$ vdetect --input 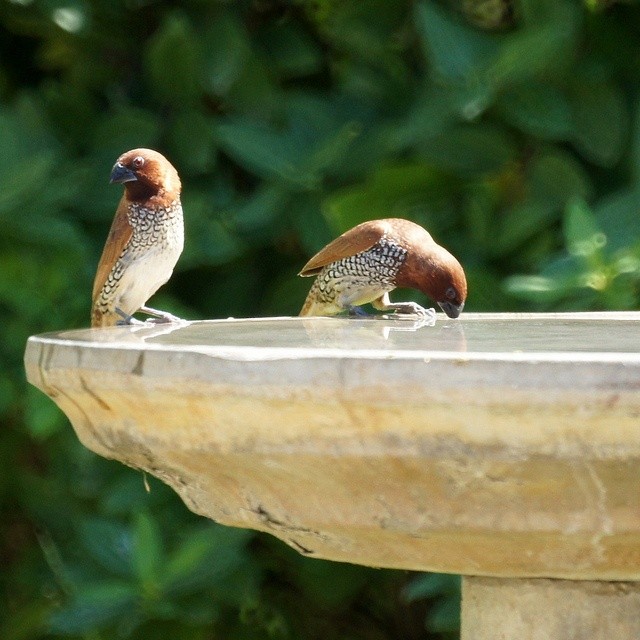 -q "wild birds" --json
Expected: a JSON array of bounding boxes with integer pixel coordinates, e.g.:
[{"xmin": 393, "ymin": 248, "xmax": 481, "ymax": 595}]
[
  {"xmin": 295, "ymin": 216, "xmax": 469, "ymax": 320},
  {"xmin": 89, "ymin": 146, "xmax": 189, "ymax": 329}
]
